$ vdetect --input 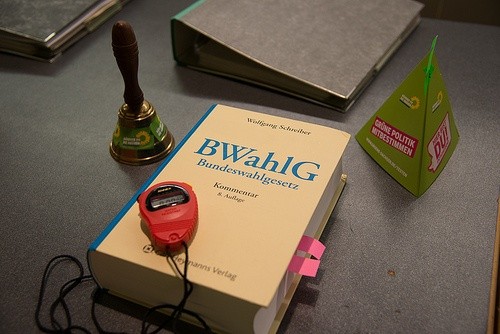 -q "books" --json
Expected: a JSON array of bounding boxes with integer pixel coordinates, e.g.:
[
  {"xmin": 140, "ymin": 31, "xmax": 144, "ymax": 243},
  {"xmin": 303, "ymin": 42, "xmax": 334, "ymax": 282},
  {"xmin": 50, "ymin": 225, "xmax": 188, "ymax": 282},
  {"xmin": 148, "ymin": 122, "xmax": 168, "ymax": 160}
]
[
  {"xmin": 169, "ymin": 1, "xmax": 426, "ymax": 112},
  {"xmin": 85, "ymin": 103, "xmax": 351, "ymax": 334},
  {"xmin": 0, "ymin": 0, "xmax": 132, "ymax": 64}
]
